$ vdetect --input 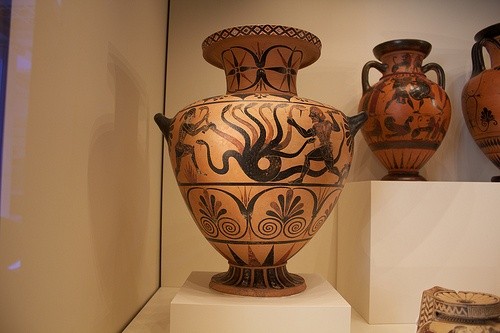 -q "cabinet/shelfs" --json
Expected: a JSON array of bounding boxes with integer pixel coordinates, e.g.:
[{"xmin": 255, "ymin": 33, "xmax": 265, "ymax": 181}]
[
  {"xmin": 336, "ymin": 178, "xmax": 500, "ymax": 326},
  {"xmin": 168, "ymin": 270, "xmax": 352, "ymax": 333}
]
